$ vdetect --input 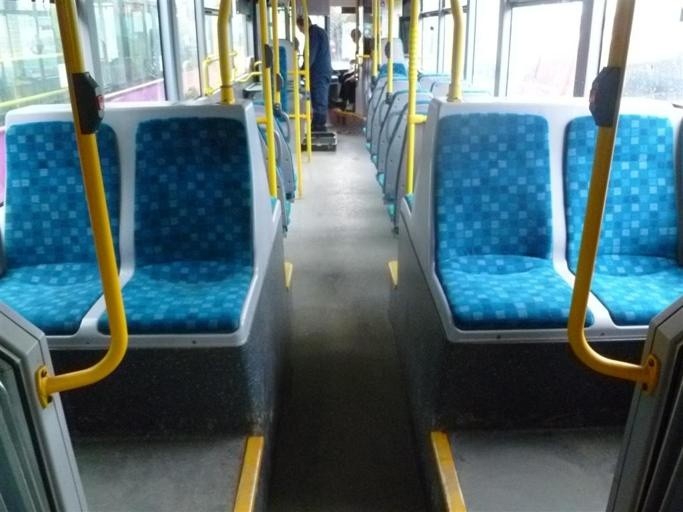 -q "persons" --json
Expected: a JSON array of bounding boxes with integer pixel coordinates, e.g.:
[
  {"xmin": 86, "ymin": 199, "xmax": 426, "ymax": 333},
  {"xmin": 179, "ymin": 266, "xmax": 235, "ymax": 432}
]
[
  {"xmin": 295, "ymin": 14, "xmax": 331, "ymax": 132},
  {"xmin": 340, "ymin": 28, "xmax": 370, "ymax": 112}
]
